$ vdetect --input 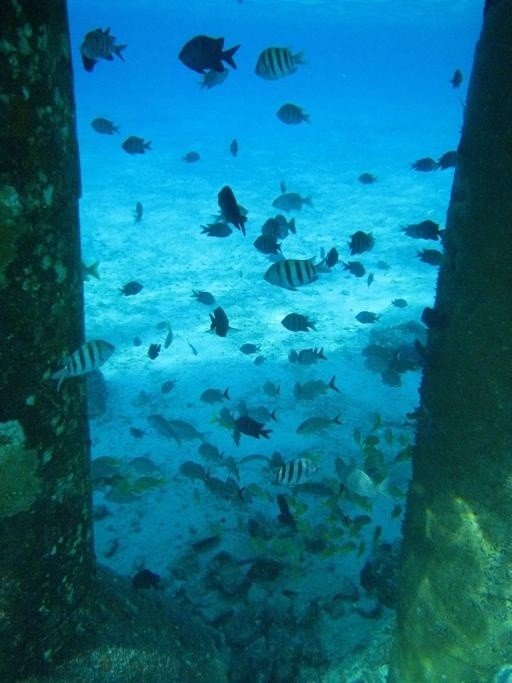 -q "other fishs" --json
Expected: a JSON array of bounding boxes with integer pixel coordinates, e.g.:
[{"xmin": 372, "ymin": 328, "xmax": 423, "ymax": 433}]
[{"xmin": 0, "ymin": 25, "xmax": 510, "ymax": 681}]
